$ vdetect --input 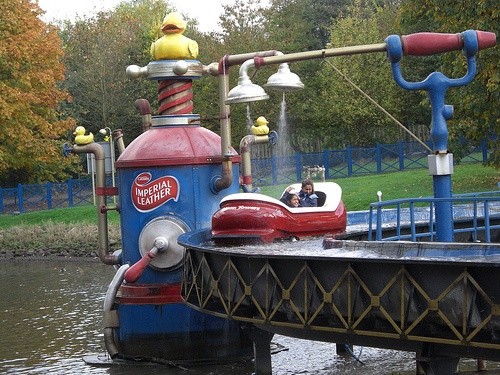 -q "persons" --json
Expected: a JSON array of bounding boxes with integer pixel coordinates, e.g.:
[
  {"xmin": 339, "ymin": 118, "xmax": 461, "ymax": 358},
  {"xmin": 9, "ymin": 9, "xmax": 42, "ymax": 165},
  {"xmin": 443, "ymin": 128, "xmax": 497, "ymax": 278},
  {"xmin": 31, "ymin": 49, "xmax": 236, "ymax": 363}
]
[
  {"xmin": 279, "ymin": 186, "xmax": 306, "ymax": 207},
  {"xmin": 298, "ymin": 178, "xmax": 319, "ymax": 208},
  {"xmin": 302, "ymin": 163, "xmax": 326, "ymax": 182}
]
